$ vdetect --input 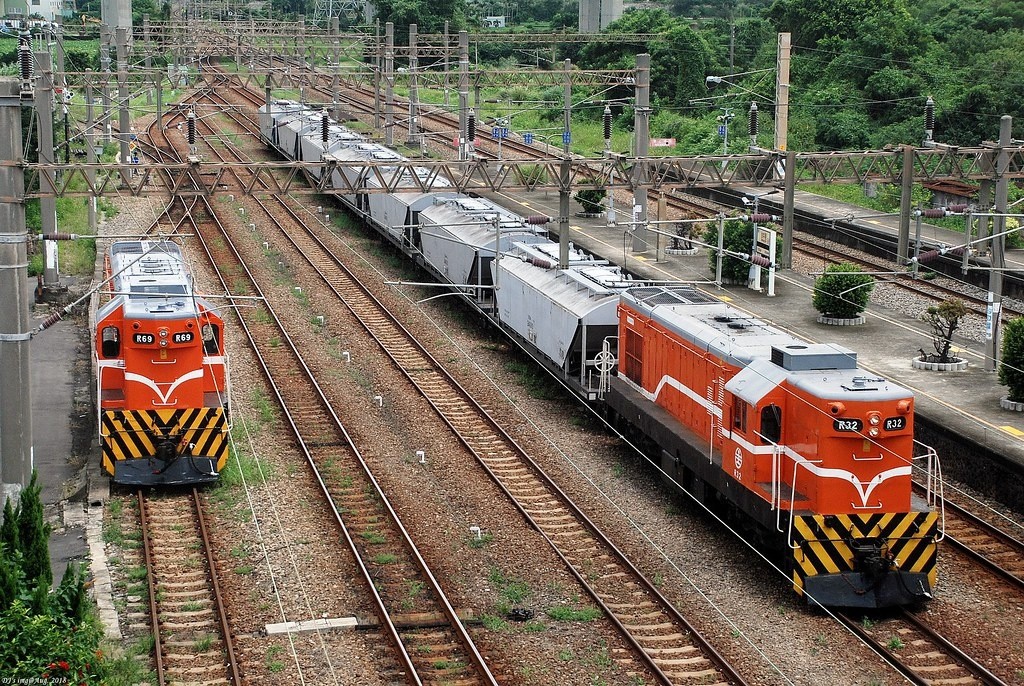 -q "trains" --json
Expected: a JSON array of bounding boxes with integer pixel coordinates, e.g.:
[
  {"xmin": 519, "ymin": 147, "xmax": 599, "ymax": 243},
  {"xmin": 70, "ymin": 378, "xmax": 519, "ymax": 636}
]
[
  {"xmin": 93, "ymin": 240, "xmax": 233, "ymax": 488},
  {"xmin": 258, "ymin": 99, "xmax": 946, "ymax": 622}
]
